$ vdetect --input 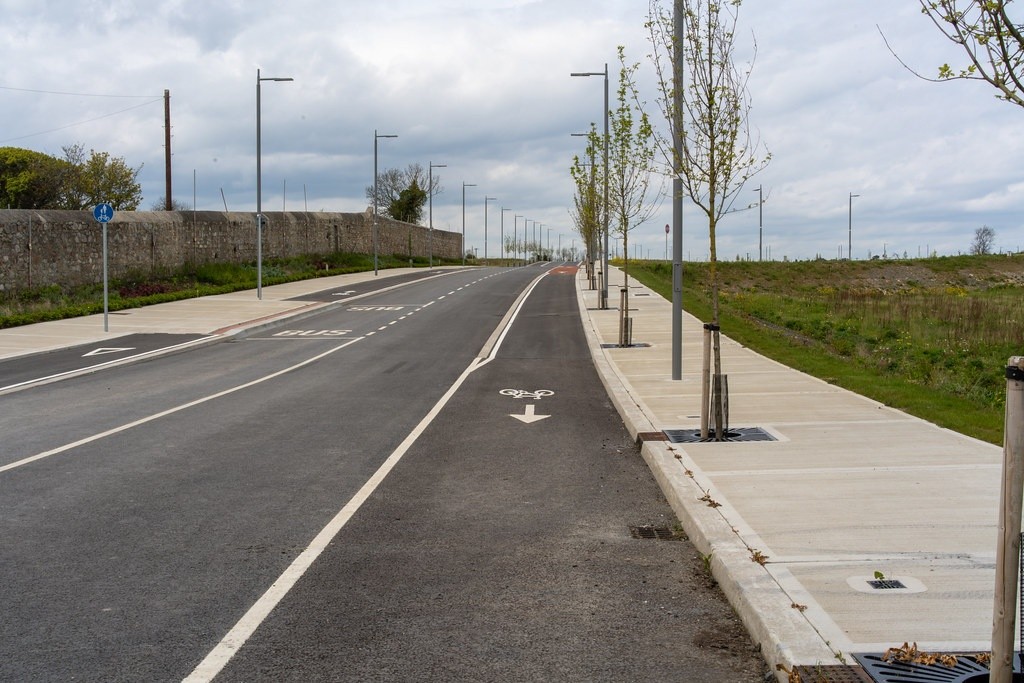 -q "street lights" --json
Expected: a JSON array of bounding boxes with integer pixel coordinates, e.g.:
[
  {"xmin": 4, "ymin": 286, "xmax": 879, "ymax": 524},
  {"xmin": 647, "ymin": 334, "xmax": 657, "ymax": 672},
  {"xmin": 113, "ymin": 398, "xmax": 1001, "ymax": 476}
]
[
  {"xmin": 501, "ymin": 206, "xmax": 512, "ymax": 265},
  {"xmin": 558, "ymin": 233, "xmax": 564, "ymax": 251},
  {"xmin": 514, "ymin": 214, "xmax": 523, "ymax": 265},
  {"xmin": 533, "ymin": 221, "xmax": 539, "ymax": 245},
  {"xmin": 539, "ymin": 224, "xmax": 547, "ymax": 249},
  {"xmin": 632, "ymin": 243, "xmax": 636, "ymax": 259},
  {"xmin": 637, "ymin": 244, "xmax": 642, "ymax": 259},
  {"xmin": 571, "ymin": 133, "xmax": 595, "ymax": 277},
  {"xmin": 429, "ymin": 161, "xmax": 448, "ymax": 269},
  {"xmin": 848, "ymin": 192, "xmax": 860, "ymax": 259},
  {"xmin": 547, "ymin": 228, "xmax": 553, "ymax": 249},
  {"xmin": 462, "ymin": 180, "xmax": 478, "ymax": 267},
  {"xmin": 374, "ymin": 129, "xmax": 398, "ymax": 276},
  {"xmin": 256, "ymin": 69, "xmax": 294, "ymax": 300},
  {"xmin": 484, "ymin": 195, "xmax": 497, "ymax": 267},
  {"xmin": 570, "ymin": 62, "xmax": 609, "ymax": 306},
  {"xmin": 525, "ymin": 218, "xmax": 533, "ymax": 263},
  {"xmin": 753, "ymin": 184, "xmax": 762, "ymax": 262}
]
